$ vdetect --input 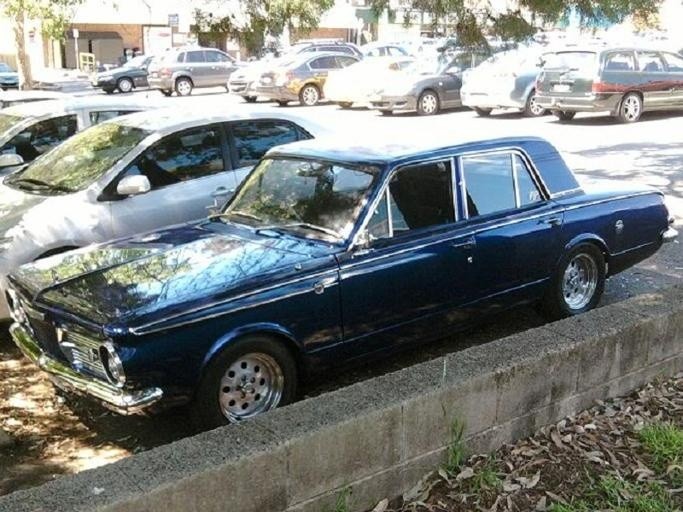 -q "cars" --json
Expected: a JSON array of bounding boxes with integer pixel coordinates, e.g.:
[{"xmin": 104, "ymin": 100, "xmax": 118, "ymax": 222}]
[
  {"xmin": 90, "ymin": 55, "xmax": 151, "ymax": 93},
  {"xmin": 0, "ymin": 62, "xmax": 19, "ymax": 90},
  {"xmin": 0, "ymin": 110, "xmax": 325, "ymax": 315},
  {"xmin": 147, "ymin": 47, "xmax": 244, "ymax": 97},
  {"xmin": 6, "ymin": 136, "xmax": 674, "ymax": 427},
  {"xmin": 0, "ymin": 98, "xmax": 158, "ymax": 176}
]
